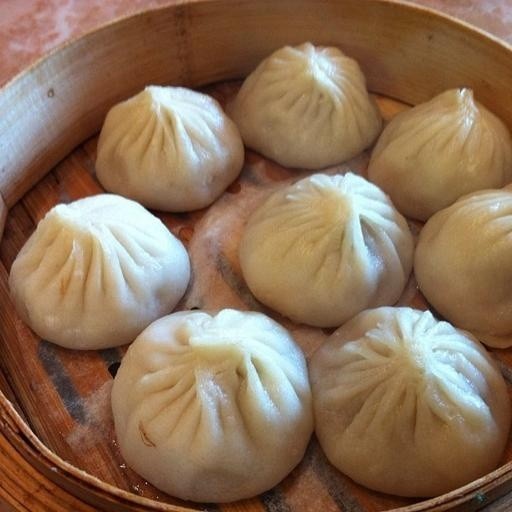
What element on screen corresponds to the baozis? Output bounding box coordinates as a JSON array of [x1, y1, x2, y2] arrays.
[[5, 39, 511, 504]]
